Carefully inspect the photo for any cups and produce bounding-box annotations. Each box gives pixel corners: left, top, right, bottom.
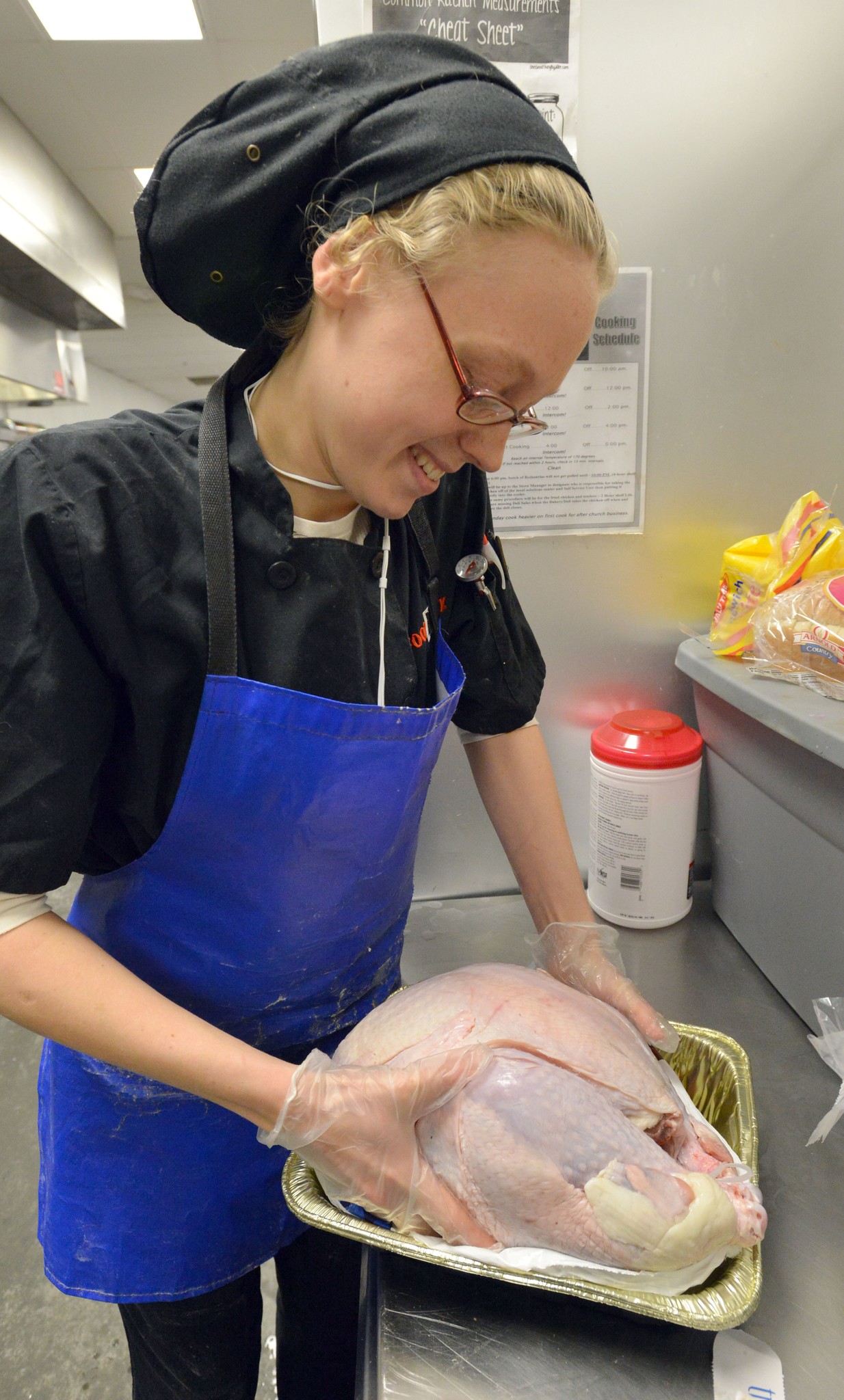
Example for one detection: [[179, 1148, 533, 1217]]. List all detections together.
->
[[584, 707, 704, 933]]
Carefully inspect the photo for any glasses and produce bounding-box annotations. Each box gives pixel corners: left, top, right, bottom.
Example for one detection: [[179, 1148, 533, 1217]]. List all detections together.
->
[[409, 253, 552, 441]]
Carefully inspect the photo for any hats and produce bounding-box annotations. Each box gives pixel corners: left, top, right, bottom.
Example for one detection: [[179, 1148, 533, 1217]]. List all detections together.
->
[[126, 30, 595, 346]]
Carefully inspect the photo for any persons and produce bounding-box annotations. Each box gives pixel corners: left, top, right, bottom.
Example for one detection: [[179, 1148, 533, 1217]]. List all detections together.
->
[[0, 30, 682, 1400]]
